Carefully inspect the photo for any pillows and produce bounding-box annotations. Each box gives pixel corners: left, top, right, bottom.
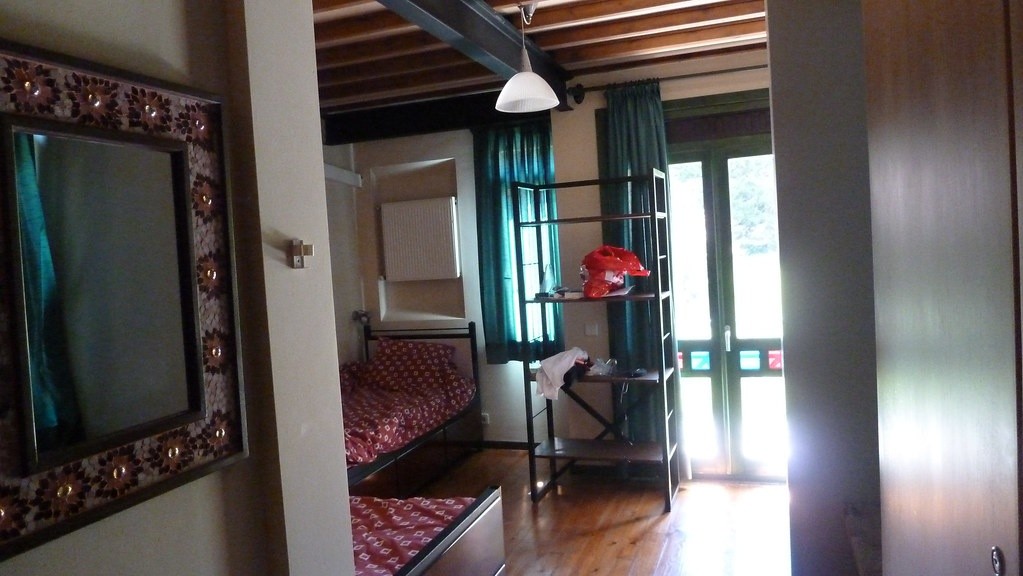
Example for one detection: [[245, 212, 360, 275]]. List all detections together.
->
[[362, 337, 458, 392]]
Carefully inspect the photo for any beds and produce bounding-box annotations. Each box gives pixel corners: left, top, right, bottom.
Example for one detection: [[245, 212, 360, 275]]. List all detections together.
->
[[339, 317, 483, 489], [348, 485, 506, 576]]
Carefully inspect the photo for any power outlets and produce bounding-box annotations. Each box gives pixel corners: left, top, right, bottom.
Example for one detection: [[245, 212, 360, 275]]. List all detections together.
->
[[481, 413, 489, 424]]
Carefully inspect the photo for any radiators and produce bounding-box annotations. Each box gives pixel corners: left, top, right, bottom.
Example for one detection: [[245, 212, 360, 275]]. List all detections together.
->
[[380, 197, 460, 281]]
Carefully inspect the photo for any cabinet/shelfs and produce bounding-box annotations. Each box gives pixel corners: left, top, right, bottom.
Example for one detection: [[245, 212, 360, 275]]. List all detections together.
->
[[509, 168, 679, 512]]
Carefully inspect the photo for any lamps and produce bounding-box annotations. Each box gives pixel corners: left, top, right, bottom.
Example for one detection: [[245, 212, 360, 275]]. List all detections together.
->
[[495, 6, 559, 113]]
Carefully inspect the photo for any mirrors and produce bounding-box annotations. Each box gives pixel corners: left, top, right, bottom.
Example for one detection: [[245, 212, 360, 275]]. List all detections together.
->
[[0, 37, 249, 563]]
